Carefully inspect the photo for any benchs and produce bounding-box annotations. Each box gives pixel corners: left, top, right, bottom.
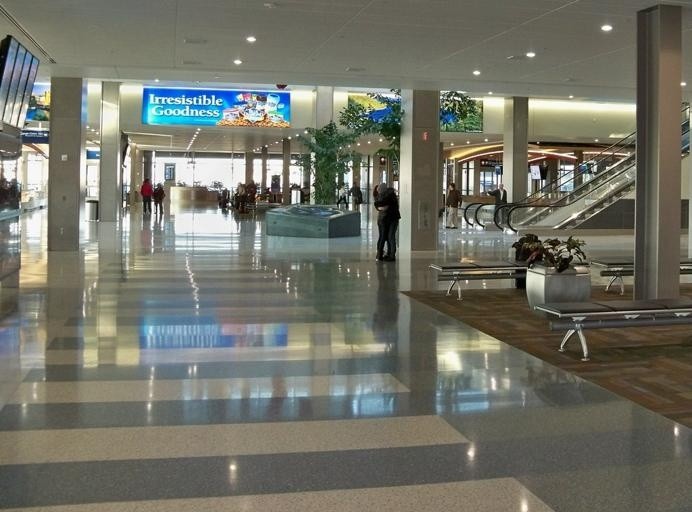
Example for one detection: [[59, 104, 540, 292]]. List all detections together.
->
[[429, 262, 532, 301], [230, 195, 283, 218], [533, 297, 692, 362], [590, 255, 692, 297]]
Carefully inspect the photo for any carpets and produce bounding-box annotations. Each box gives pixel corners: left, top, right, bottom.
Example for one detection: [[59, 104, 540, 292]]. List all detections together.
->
[[399, 283, 692, 429]]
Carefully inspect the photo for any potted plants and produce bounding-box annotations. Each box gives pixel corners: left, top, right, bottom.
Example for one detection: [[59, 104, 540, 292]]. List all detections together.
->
[[520, 235, 590, 311]]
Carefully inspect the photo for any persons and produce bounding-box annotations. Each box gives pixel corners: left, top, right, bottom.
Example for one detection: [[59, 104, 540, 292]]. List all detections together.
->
[[245, 179, 257, 199], [445, 183, 462, 229], [235, 182, 247, 211], [373, 184, 395, 262], [487, 184, 507, 224], [152, 183, 166, 214], [374, 182, 400, 262], [348, 182, 361, 212], [336, 182, 349, 207], [141, 178, 154, 215]]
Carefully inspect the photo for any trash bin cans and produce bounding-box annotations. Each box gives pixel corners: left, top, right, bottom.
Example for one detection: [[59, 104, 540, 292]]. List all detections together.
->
[[85, 200, 97, 221]]
